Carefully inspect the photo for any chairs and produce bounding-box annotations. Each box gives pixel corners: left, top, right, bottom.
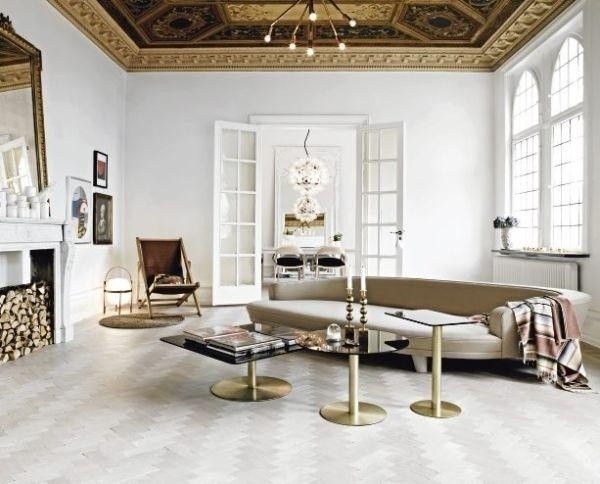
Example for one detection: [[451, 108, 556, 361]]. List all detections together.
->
[[272, 245, 304, 282], [136, 237, 202, 319], [312, 245, 347, 280]]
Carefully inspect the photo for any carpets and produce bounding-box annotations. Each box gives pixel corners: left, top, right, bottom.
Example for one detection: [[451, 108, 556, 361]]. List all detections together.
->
[[100, 312, 184, 329]]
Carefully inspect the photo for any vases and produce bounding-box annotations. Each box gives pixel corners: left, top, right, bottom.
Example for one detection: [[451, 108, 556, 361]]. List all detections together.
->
[[501, 230, 510, 250]]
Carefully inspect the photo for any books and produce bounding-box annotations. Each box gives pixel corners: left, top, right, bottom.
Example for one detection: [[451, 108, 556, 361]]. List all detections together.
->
[[184, 325, 308, 357]]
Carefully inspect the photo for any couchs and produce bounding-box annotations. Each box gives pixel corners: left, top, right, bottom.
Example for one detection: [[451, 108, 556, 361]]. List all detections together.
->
[[247, 276, 590, 372]]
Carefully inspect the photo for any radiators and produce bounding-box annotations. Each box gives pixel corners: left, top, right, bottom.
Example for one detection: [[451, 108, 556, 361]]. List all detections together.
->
[[492, 257, 578, 291]]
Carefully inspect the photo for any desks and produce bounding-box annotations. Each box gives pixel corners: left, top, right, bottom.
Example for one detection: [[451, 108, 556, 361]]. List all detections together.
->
[[386, 309, 479, 418], [296, 328, 410, 426]]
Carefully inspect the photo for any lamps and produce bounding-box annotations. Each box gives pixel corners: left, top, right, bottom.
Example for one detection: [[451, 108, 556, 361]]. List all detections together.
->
[[264, 1, 356, 56]]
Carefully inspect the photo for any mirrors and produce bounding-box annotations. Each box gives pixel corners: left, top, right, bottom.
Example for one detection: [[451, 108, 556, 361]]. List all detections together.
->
[[0, 27, 51, 217]]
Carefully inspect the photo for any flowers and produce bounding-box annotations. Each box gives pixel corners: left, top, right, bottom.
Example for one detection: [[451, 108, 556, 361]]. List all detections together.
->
[[494, 215, 519, 229]]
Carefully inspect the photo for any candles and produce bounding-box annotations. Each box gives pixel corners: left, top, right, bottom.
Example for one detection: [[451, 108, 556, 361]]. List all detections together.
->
[[346, 267, 352, 288], [360, 265, 366, 290]]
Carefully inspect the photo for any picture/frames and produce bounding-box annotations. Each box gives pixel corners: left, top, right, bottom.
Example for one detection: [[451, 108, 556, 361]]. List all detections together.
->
[[273, 145, 338, 249], [64, 177, 92, 243], [93, 192, 113, 244], [93, 150, 108, 188]]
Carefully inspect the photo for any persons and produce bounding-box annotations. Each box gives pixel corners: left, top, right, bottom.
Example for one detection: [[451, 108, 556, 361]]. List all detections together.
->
[[98, 202, 107, 235], [77, 197, 88, 237]]
